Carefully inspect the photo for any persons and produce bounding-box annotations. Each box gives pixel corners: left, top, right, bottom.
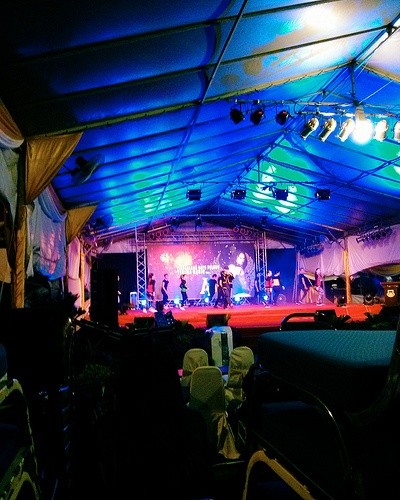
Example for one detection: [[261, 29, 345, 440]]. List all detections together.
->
[[180, 274, 189, 310], [208, 266, 234, 310], [147, 273, 157, 312], [264, 269, 287, 304], [295, 267, 308, 304], [161, 274, 169, 307], [254, 272, 261, 305], [314, 268, 325, 306]]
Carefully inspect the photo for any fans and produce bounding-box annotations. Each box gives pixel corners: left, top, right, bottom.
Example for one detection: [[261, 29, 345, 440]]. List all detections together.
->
[[76, 154, 103, 184]]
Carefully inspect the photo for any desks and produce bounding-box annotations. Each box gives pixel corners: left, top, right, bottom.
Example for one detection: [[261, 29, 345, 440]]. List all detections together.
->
[[243, 331, 397, 472]]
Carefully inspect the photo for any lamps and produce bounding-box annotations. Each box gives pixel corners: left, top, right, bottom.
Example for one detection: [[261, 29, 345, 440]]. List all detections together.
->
[[183, 181, 331, 201], [231, 100, 400, 143]]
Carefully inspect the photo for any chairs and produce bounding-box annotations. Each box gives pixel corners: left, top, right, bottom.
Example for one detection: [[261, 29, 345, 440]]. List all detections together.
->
[[179, 332, 255, 460]]
